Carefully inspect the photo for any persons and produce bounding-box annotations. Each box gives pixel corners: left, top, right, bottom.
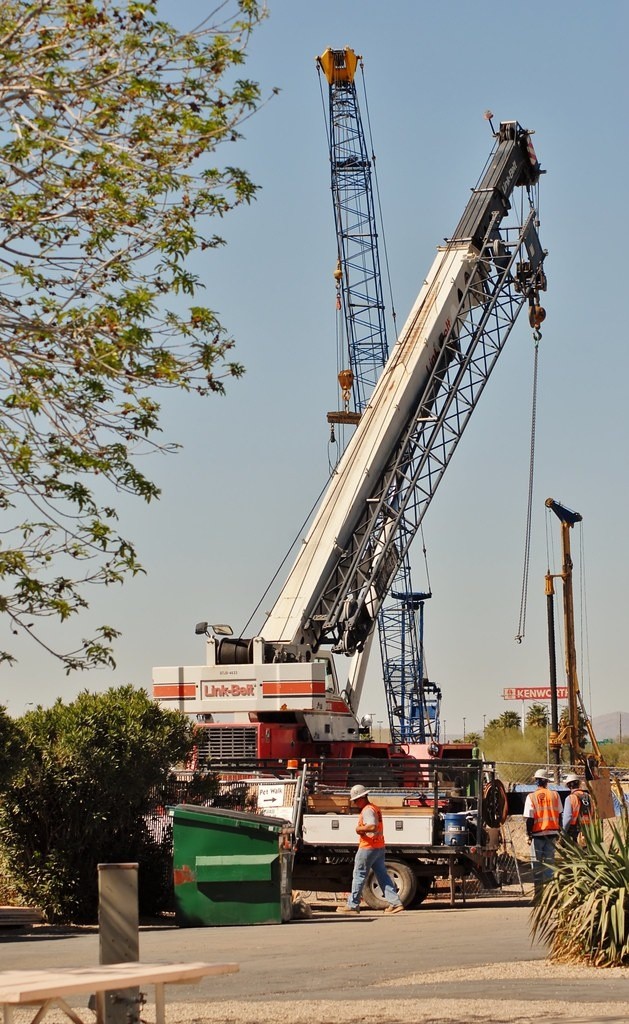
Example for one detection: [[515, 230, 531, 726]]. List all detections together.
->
[[336, 784, 404, 914], [523, 769, 564, 895], [557, 773, 596, 847]]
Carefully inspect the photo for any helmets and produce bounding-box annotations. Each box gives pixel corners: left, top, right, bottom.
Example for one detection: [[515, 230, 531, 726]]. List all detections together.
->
[[566, 775, 579, 784], [534, 769, 548, 779], [427, 741, 439, 756], [350, 785, 371, 801]]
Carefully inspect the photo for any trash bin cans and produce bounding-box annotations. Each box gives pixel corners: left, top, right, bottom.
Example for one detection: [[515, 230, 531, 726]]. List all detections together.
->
[[167, 799, 297, 925]]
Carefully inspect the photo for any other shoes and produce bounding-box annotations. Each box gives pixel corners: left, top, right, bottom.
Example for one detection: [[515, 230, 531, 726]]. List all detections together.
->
[[336, 906, 360, 914], [385, 905, 403, 914]]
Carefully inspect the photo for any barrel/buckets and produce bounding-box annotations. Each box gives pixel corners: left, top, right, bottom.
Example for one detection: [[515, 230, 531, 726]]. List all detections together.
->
[[445, 813, 470, 846]]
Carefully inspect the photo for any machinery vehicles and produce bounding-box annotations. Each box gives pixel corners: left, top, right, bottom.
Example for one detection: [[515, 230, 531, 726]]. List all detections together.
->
[[134, 42, 625, 911]]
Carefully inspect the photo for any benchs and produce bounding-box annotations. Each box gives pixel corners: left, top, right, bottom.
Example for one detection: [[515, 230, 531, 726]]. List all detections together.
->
[[0, 960, 240, 1024]]
